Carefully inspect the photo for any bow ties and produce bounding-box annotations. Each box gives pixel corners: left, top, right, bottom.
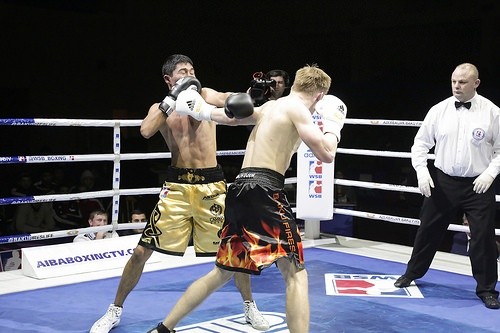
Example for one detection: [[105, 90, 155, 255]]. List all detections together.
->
[[455, 101, 471, 109]]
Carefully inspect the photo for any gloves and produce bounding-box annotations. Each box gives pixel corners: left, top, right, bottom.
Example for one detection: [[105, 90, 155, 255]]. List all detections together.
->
[[472, 167, 499, 194], [416, 167, 434, 197]]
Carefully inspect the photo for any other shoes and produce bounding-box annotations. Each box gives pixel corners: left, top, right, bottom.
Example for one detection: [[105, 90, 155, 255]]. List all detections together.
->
[[394, 272, 414, 288], [476, 289, 500, 309]]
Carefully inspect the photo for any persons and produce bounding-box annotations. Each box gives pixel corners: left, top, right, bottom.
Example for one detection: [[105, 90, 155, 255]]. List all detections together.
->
[[394, 63, 500, 309], [73, 211, 119, 242], [128, 209, 147, 234], [90, 56, 270, 333], [453, 213, 471, 257], [147, 66, 347, 333], [9, 169, 105, 232], [245, 69, 288, 100]]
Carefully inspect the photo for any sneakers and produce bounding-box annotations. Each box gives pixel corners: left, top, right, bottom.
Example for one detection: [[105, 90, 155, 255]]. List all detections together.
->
[[90, 304, 123, 333], [243, 300, 269, 331]]
